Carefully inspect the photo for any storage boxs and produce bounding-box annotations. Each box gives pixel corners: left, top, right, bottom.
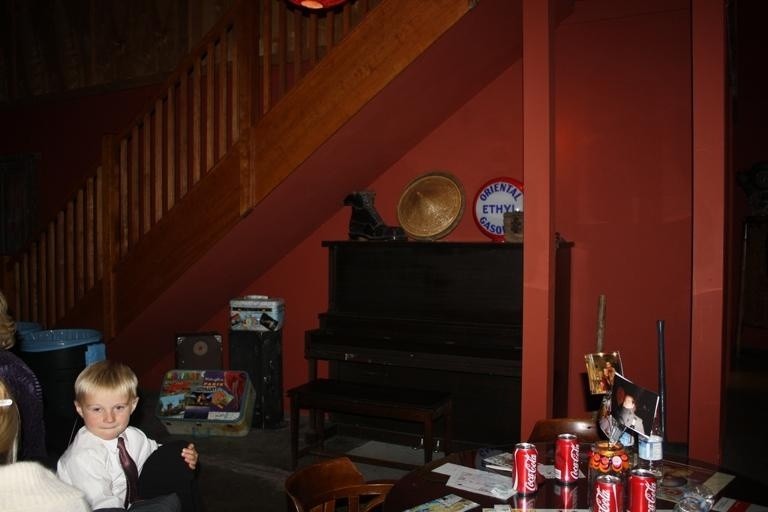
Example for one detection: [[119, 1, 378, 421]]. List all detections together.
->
[[155, 369, 257, 438], [230, 294, 285, 332]]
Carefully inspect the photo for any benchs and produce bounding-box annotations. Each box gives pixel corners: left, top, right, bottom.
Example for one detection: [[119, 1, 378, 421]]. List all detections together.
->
[[286, 378, 456, 471]]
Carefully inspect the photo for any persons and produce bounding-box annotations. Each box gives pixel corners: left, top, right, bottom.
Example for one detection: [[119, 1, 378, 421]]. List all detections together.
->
[[56, 357, 202, 512], [617, 392, 646, 435], [0, 376, 94, 512], [1, 291, 50, 460], [602, 360, 616, 391]]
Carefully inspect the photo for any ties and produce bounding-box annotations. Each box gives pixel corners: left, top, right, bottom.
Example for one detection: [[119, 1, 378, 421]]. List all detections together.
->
[[117, 437, 140, 509]]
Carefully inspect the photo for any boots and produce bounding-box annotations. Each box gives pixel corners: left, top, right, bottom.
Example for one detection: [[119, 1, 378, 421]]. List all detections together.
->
[[343, 191, 408, 241]]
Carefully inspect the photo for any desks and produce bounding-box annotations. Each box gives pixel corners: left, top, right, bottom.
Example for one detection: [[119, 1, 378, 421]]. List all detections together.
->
[[382, 440, 768, 512]]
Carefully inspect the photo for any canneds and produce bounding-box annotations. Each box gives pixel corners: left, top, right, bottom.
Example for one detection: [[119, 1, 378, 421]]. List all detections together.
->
[[592, 475, 624, 512], [512, 442, 538, 497], [554, 434, 580, 485], [626, 469, 656, 512]]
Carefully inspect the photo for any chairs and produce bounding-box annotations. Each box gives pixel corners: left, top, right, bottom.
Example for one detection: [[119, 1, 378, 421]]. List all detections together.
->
[[285, 457, 396, 512]]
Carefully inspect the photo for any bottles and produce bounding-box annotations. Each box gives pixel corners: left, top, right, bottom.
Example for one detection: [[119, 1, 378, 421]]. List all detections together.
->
[[638, 417, 665, 483]]
